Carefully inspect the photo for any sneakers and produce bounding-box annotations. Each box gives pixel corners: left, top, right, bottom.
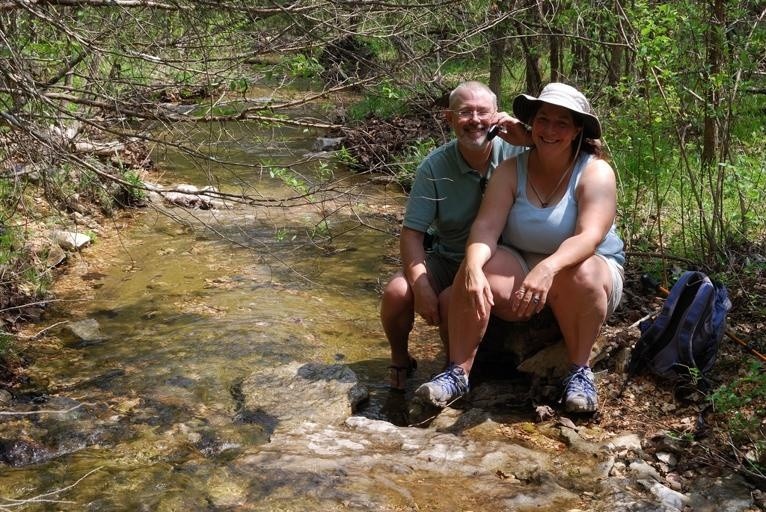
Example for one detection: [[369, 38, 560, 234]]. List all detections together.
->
[[563, 364, 598, 412], [413, 366, 471, 407], [388, 358, 417, 387]]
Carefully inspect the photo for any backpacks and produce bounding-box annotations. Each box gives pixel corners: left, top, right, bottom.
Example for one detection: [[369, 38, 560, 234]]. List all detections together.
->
[[630, 271, 734, 398]]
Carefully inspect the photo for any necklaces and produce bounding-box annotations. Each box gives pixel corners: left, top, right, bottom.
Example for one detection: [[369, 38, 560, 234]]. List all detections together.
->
[[460, 141, 493, 197]]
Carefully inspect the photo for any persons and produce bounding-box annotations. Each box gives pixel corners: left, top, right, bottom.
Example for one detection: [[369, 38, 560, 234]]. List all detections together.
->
[[374, 81, 539, 402], [414, 80, 627, 414]]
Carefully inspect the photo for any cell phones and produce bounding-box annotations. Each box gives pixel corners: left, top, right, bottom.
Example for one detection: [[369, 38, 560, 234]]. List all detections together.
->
[[487, 124, 502, 141]]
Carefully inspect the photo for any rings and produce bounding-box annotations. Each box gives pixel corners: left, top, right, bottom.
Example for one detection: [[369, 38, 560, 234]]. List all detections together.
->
[[533, 296, 540, 305], [433, 320, 439, 324], [520, 287, 526, 296]]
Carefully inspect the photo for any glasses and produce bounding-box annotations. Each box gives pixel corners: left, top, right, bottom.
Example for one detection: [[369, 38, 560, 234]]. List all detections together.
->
[[450, 109, 495, 119]]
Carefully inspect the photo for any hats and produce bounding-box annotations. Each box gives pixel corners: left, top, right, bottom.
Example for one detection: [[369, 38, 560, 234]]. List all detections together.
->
[[514, 82, 602, 139]]
